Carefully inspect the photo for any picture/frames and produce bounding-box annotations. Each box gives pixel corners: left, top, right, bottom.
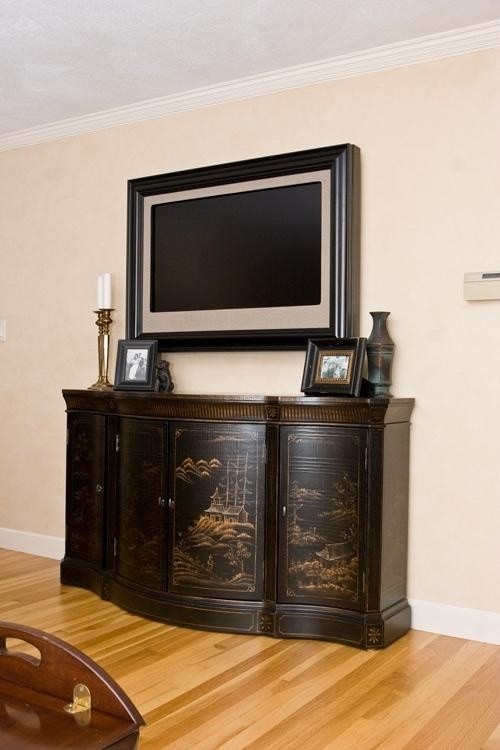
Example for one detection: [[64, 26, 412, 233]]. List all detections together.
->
[[113, 339, 160, 392], [124, 143, 360, 351], [301, 336, 368, 398]]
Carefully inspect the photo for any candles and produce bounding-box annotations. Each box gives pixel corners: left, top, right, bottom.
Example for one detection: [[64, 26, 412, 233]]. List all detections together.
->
[[95, 271, 112, 311]]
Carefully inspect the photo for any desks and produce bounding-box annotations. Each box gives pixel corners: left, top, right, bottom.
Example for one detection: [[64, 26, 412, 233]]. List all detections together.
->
[[0, 620, 146, 750]]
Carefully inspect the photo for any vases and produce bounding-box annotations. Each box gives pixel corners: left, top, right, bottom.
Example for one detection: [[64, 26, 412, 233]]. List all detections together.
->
[[367, 311, 396, 398]]
